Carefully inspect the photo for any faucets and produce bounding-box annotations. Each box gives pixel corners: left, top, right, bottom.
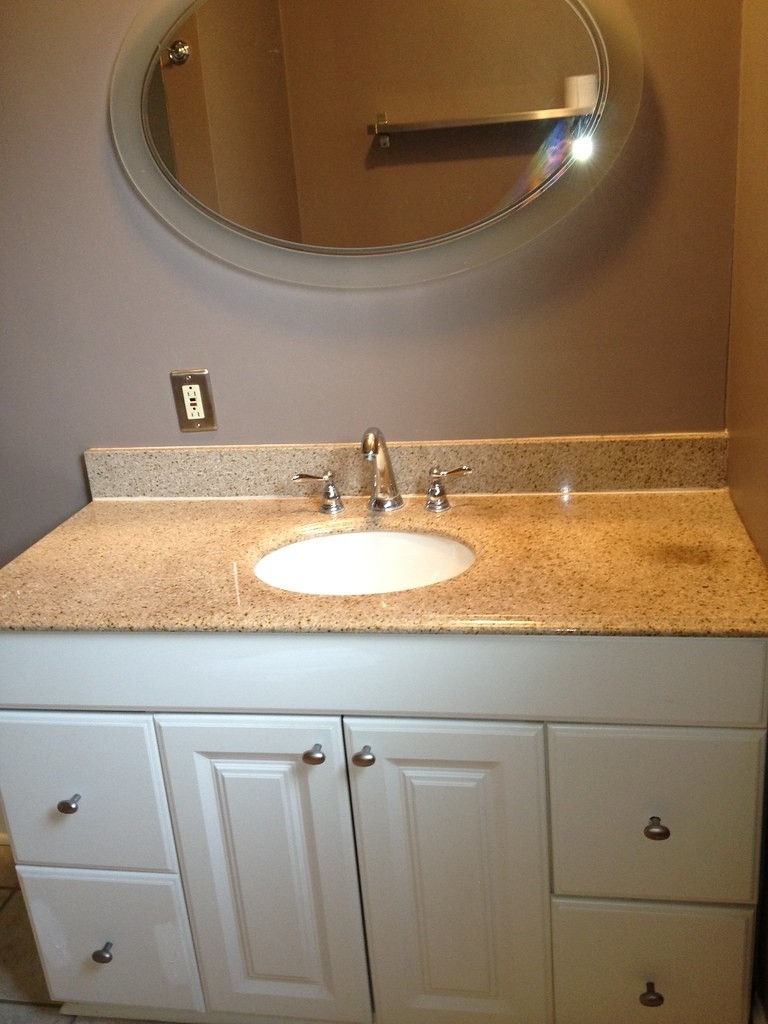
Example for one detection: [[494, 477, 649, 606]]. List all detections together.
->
[[361, 426, 404, 511]]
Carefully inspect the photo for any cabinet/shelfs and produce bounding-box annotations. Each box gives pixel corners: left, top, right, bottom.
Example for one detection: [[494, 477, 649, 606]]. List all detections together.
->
[[0, 628, 207, 1024], [154, 632, 556, 1024], [543, 633, 768, 1024]]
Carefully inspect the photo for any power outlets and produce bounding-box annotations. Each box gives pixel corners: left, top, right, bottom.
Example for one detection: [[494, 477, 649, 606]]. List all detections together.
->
[[170, 368, 218, 433]]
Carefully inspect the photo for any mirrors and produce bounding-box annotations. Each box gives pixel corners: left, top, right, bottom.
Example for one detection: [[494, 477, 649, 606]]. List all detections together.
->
[[109, 0, 645, 289]]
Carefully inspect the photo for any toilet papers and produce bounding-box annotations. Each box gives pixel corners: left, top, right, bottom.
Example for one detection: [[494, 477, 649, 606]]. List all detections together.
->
[[564, 74, 597, 108]]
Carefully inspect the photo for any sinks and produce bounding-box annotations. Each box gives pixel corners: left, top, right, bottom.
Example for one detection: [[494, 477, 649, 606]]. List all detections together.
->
[[254, 531, 475, 597]]
